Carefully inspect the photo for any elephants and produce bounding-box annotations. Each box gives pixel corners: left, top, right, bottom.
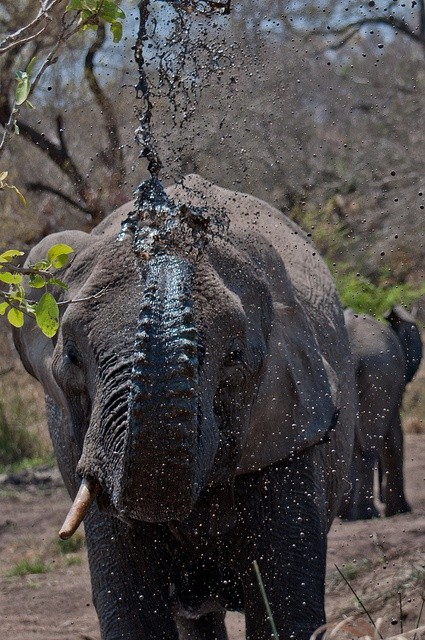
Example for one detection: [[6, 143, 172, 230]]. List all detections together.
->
[[339, 306, 423, 520], [8, 174, 356, 640]]
